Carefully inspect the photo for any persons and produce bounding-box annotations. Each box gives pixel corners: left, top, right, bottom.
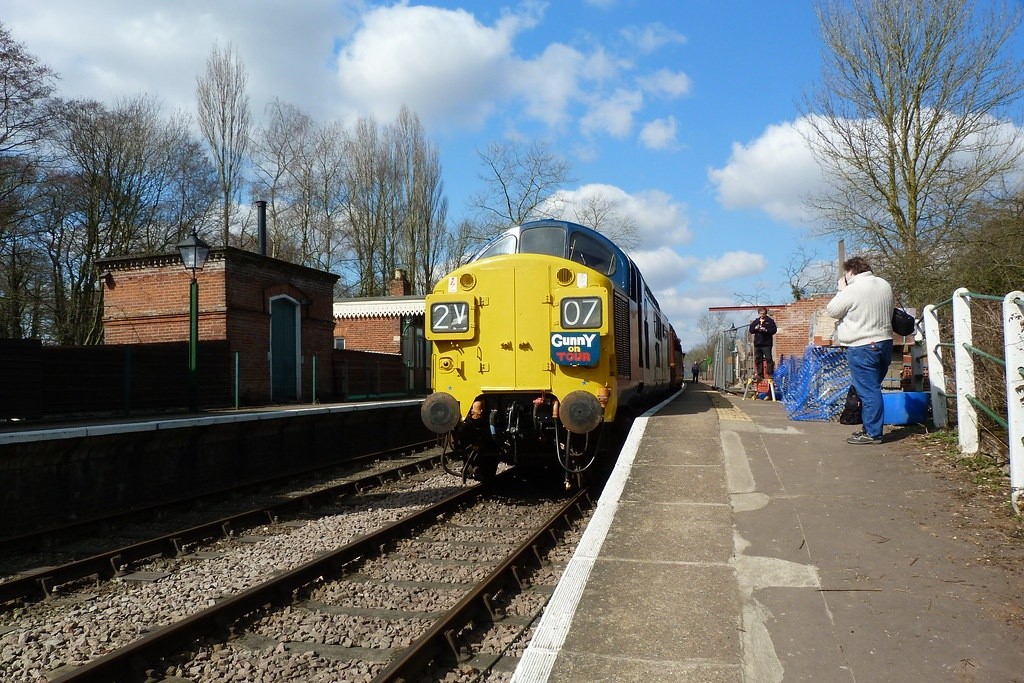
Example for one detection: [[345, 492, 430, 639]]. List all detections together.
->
[[828, 257, 894, 443], [692, 360, 699, 384], [749, 307, 777, 378]]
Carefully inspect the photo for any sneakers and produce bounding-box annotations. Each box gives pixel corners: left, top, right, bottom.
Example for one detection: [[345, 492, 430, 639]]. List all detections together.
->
[[852, 430, 868, 436], [848, 434, 882, 444]]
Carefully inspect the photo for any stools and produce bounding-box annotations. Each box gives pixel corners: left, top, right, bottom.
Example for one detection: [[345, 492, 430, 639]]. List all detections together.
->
[[742, 378, 778, 402]]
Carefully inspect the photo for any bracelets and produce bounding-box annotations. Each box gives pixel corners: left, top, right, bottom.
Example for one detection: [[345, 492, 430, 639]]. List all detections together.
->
[[766, 330, 767, 332]]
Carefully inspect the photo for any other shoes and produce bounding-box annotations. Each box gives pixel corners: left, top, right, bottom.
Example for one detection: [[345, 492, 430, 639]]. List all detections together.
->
[[753, 375, 763, 380], [769, 374, 774, 379]]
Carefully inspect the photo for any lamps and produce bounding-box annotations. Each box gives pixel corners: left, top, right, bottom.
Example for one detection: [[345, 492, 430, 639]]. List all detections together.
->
[[99, 271, 110, 283]]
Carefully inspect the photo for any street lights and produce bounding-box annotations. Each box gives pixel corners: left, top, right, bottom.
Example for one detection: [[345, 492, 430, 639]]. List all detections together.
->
[[727, 323, 737, 387], [176, 225, 212, 416]]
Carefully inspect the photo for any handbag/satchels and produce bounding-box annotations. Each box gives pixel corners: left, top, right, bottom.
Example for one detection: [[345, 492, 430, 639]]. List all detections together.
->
[[891, 308, 915, 336]]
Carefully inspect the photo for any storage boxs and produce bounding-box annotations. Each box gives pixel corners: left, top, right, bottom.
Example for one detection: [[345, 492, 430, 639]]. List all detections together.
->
[[881, 392, 931, 425], [881, 350, 904, 388]]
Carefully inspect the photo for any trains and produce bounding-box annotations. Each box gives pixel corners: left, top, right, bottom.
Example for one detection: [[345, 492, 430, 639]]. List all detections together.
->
[[422, 219, 685, 491]]
